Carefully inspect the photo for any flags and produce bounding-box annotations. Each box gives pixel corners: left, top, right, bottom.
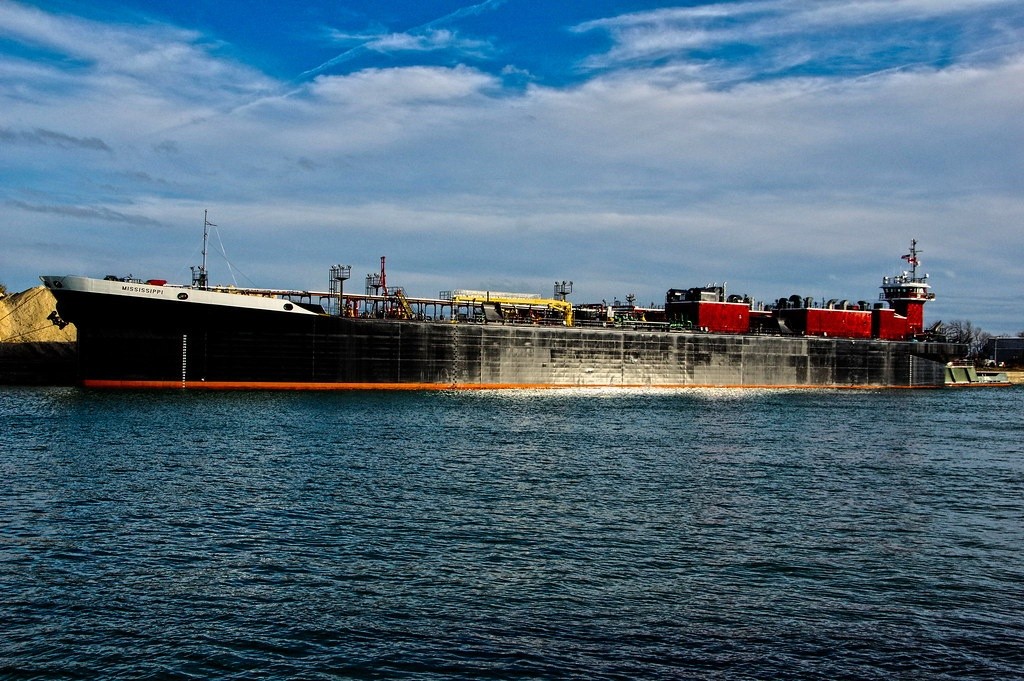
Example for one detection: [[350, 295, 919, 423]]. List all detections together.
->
[[901, 254, 916, 263]]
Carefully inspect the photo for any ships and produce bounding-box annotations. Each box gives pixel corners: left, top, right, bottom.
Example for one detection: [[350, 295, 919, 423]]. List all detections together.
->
[[39, 205, 969, 391]]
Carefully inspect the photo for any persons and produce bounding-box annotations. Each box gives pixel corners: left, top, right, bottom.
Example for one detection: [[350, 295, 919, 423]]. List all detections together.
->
[[348, 304, 354, 317], [946, 356, 968, 366], [508, 308, 565, 326], [360, 311, 398, 320]]
